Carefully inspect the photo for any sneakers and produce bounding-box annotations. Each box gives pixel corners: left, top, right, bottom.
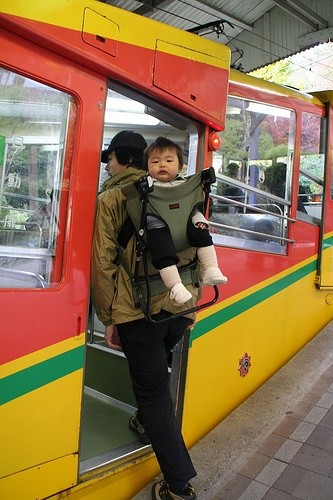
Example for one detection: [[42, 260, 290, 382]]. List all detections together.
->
[[151, 479, 197, 500], [129, 410, 151, 445]]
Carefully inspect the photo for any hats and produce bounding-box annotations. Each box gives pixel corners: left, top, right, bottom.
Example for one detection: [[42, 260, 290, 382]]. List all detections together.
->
[[101, 131, 147, 163]]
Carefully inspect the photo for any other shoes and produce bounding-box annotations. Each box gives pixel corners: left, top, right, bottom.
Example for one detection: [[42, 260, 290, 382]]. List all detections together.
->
[[170, 283, 192, 303], [203, 267, 227, 285]]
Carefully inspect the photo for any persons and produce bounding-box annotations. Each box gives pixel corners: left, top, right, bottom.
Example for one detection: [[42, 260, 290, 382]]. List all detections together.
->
[[263, 163, 307, 214], [25, 190, 60, 250], [91, 130, 204, 500], [218, 163, 246, 205], [143, 136, 227, 304]]
